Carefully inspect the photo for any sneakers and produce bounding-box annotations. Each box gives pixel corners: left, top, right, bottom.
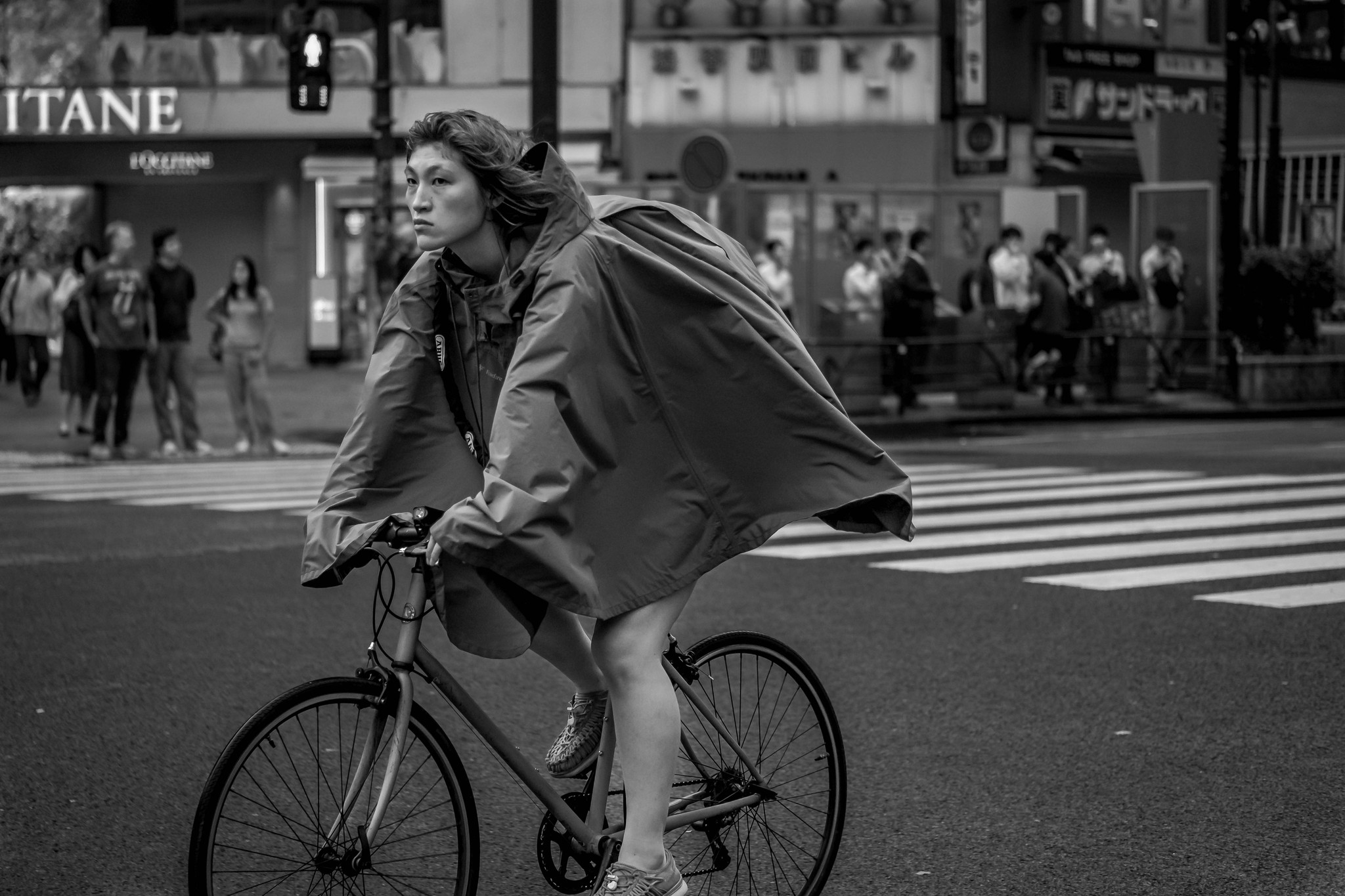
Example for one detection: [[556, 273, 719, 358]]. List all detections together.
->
[[593, 849, 688, 896], [545, 688, 608, 778]]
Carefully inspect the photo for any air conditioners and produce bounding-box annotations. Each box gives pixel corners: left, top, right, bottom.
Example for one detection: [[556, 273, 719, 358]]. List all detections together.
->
[[956, 116, 1006, 161]]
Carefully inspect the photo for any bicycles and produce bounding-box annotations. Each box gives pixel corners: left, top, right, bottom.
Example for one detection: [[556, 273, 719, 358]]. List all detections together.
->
[[189, 505, 848, 896]]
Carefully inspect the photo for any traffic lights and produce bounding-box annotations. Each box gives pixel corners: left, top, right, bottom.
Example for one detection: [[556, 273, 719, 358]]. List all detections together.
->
[[289, 29, 334, 115]]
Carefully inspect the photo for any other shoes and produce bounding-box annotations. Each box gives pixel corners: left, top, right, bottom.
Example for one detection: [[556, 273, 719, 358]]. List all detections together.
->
[[24, 392, 39, 403], [77, 425, 91, 435], [897, 399, 929, 413], [194, 439, 214, 454], [89, 443, 112, 461], [59, 422, 69, 437], [236, 438, 250, 453], [164, 439, 176, 455], [112, 445, 139, 459], [1043, 395, 1084, 407], [272, 439, 291, 454]]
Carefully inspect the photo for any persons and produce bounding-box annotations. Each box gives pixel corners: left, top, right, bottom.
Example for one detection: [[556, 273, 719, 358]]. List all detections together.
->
[[0, 219, 211, 460], [202, 254, 294, 457], [1140, 225, 1188, 391], [756, 223, 1127, 418], [299, 109, 729, 896]]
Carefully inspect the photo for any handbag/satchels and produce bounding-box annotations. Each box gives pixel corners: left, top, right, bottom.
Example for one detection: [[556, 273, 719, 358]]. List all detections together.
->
[[208, 286, 229, 361]]
